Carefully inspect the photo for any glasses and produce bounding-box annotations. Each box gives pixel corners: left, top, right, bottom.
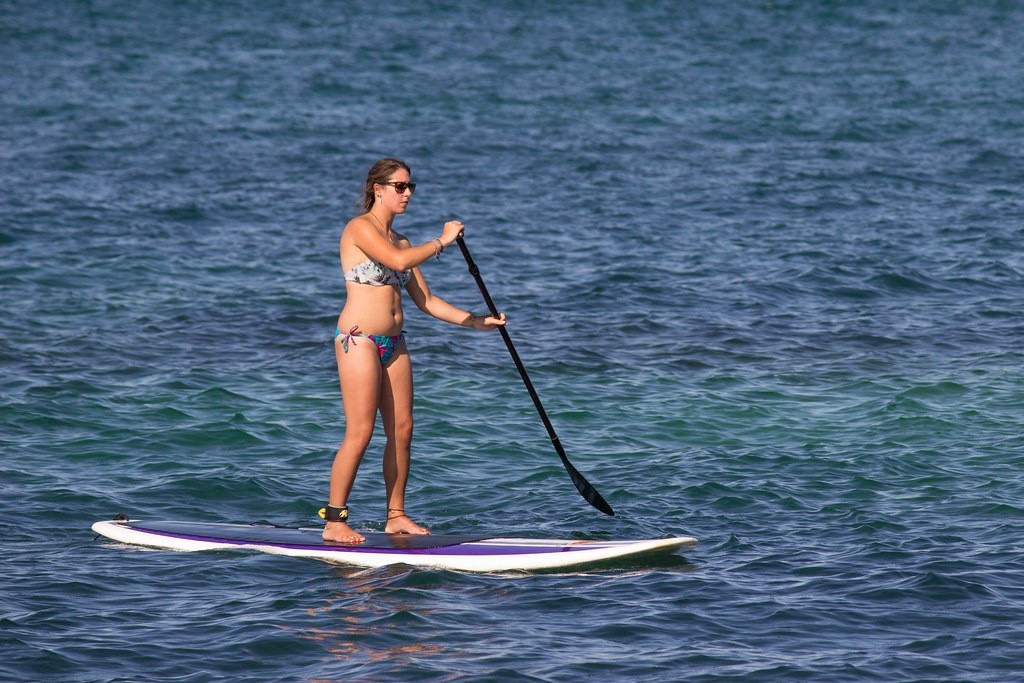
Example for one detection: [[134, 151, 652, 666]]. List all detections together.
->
[[378, 181, 416, 194]]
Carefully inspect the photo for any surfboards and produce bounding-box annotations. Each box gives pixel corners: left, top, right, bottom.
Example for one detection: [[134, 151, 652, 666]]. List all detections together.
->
[[88, 515, 700, 574]]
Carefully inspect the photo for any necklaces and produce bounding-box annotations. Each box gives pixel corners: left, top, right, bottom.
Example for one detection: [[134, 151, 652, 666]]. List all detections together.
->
[[369, 211, 394, 244]]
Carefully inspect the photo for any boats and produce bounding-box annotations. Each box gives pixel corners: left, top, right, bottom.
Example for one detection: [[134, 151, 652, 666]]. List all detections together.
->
[[92, 513, 699, 570]]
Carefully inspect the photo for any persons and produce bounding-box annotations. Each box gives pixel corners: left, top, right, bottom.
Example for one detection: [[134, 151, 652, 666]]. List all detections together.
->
[[320, 156, 508, 544]]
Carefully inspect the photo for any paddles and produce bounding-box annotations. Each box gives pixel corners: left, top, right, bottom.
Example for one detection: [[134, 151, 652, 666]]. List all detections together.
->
[[456, 233, 617, 517]]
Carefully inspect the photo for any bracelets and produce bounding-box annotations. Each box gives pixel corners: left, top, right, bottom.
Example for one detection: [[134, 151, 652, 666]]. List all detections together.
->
[[436, 238, 444, 253], [431, 240, 441, 259], [471, 315, 476, 328]]
